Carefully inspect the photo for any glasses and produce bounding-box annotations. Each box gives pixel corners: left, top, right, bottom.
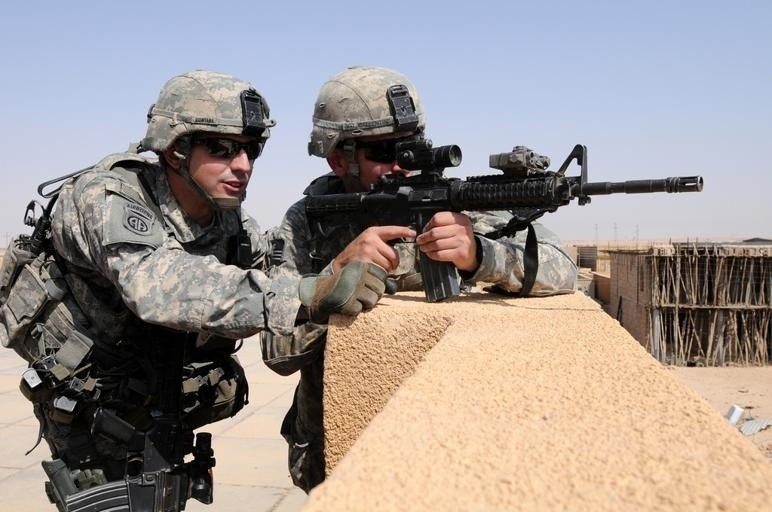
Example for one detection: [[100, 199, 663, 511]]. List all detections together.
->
[[190, 136, 266, 160], [336, 127, 424, 164]]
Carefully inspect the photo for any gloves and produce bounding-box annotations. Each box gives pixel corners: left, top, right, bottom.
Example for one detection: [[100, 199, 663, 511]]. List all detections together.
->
[[298, 262, 398, 323]]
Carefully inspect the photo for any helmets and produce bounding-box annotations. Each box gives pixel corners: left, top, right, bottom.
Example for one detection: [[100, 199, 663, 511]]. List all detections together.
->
[[308, 67, 425, 157], [140, 70, 277, 153]]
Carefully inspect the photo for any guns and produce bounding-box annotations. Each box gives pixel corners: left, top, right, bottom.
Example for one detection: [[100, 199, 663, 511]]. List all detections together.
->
[[305, 136, 703, 303], [65, 313, 215, 512]]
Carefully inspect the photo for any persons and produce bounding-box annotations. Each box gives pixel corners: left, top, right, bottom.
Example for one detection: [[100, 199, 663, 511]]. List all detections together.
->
[[258, 65, 581, 495], [2, 69, 399, 512]]
